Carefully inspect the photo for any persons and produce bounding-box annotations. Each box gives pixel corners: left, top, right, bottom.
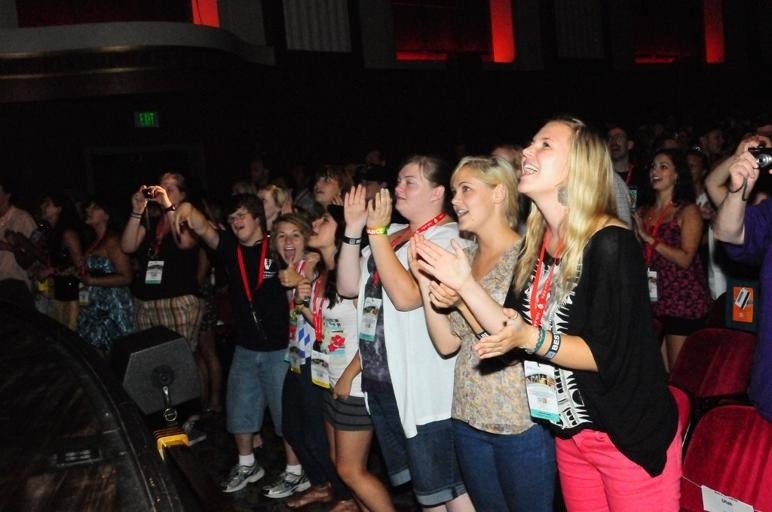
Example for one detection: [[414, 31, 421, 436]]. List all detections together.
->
[[652, 125, 772, 208], [408, 156, 557, 511], [0, 187, 38, 299], [603, 123, 646, 230], [217, 151, 409, 224], [170, 192, 311, 497], [195, 203, 225, 415], [336, 156, 477, 512], [33, 192, 84, 332], [711, 139, 772, 428], [270, 214, 359, 512], [296, 205, 395, 512], [412, 119, 682, 507], [77, 195, 139, 361], [224, 151, 398, 233], [632, 151, 711, 374], [488, 144, 532, 237], [121, 172, 207, 354], [687, 153, 731, 329]]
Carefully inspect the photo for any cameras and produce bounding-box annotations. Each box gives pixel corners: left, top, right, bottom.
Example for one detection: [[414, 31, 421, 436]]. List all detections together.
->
[[750, 146, 768, 168], [143, 188, 159, 199]]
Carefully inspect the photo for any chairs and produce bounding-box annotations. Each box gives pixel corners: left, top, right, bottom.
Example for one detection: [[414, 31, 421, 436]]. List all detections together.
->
[[680, 405, 772, 512], [668, 385, 691, 448], [667, 327, 755, 397]]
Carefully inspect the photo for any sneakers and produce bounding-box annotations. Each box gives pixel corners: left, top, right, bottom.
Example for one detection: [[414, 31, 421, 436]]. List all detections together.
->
[[264, 470, 311, 498], [220, 462, 264, 492]]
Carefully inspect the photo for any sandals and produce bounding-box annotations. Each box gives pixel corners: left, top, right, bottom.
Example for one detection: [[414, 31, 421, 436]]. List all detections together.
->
[[328, 499, 359, 512], [285, 484, 332, 507]]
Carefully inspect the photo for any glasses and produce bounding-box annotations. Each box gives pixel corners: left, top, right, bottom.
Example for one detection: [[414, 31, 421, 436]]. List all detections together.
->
[[226, 213, 252, 224]]
